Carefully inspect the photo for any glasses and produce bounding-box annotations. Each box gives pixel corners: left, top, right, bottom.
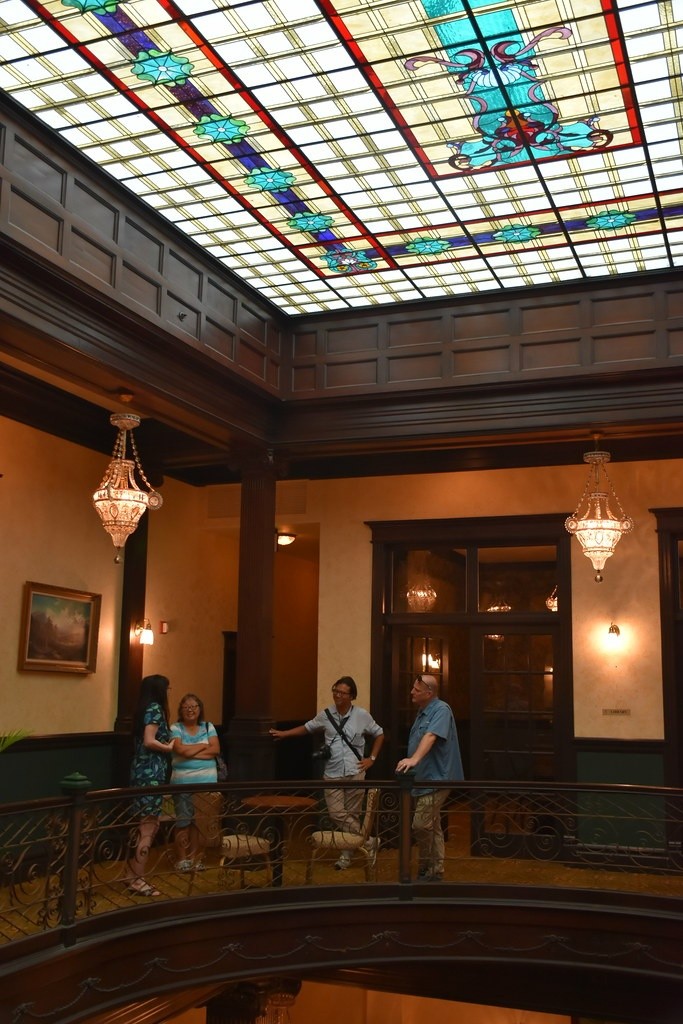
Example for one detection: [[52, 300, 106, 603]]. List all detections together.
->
[[415, 674, 429, 687], [332, 688, 351, 696], [182, 705, 200, 710], [167, 687, 173, 693]]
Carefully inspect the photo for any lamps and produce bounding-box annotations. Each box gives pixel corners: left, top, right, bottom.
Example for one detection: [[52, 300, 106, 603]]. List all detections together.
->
[[563, 431, 634, 584], [134, 618, 154, 645], [544, 585, 557, 611], [91, 394, 164, 563], [605, 623, 620, 651], [407, 551, 437, 612], [485, 593, 512, 612], [278, 533, 297, 545]]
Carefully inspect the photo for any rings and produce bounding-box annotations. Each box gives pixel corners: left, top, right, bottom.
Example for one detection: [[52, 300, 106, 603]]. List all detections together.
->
[[403, 764, 406, 767]]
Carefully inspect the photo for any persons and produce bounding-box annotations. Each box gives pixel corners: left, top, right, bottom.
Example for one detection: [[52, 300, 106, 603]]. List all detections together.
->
[[166, 694, 221, 872], [396, 674, 464, 880], [128, 674, 176, 896], [269, 675, 385, 870]]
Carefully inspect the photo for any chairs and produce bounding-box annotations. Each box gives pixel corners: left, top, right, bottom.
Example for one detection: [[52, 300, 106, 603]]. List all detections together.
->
[[304, 788, 380, 884], [186, 792, 270, 897]]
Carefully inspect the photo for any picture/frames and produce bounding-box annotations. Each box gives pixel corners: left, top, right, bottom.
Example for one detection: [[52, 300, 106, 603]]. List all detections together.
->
[[17, 580, 102, 674]]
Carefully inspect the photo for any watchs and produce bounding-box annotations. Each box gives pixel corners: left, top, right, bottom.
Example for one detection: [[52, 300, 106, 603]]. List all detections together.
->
[[370, 756, 375, 761]]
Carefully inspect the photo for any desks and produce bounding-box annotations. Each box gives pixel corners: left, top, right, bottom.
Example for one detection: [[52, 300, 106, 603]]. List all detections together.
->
[[240, 796, 319, 886]]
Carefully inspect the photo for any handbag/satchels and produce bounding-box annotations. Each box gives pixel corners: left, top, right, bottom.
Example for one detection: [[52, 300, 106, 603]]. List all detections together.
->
[[205, 721, 228, 780]]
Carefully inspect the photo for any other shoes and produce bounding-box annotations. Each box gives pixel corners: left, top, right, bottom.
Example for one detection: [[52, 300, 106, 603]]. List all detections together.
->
[[417, 868, 431, 881], [335, 856, 349, 868], [433, 874, 443, 881], [360, 836, 381, 868], [179, 858, 204, 871]]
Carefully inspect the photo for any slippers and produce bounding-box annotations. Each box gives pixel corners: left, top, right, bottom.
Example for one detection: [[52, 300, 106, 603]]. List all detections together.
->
[[128, 885, 161, 896]]
